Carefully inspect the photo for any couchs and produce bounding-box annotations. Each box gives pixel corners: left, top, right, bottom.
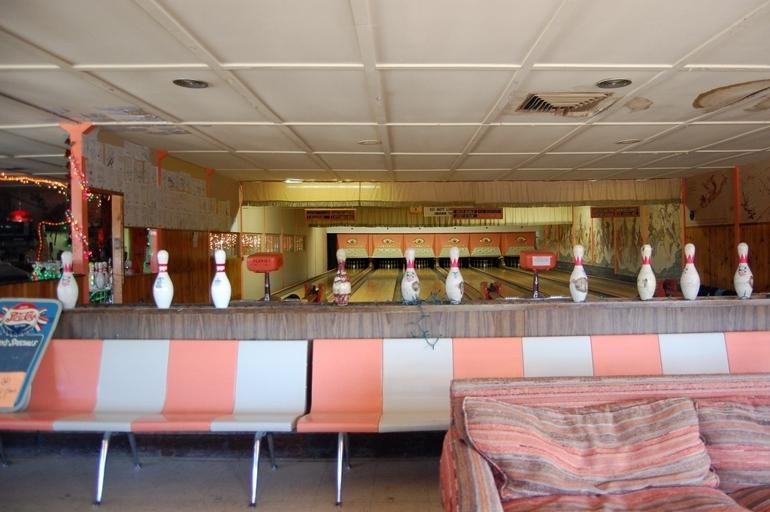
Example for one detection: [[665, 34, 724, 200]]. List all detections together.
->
[[439, 373, 770, 512]]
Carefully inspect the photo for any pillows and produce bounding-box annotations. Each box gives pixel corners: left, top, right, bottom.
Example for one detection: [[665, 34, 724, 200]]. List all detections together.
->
[[695, 397, 769, 494], [463, 396, 720, 499]]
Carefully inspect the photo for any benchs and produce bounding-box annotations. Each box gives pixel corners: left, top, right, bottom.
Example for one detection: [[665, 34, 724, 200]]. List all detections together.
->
[[0, 340, 308, 507], [296, 331, 770, 504]]
